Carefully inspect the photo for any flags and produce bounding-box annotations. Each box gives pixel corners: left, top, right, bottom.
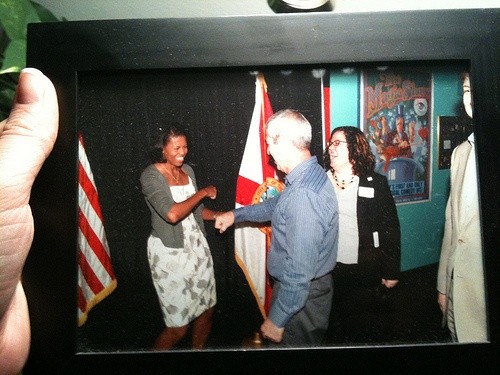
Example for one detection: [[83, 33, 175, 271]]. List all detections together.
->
[[77, 131, 118, 326], [235, 75, 289, 321]]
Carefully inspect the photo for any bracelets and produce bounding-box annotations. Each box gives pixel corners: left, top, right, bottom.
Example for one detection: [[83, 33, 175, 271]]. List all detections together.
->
[[213, 211, 218, 221]]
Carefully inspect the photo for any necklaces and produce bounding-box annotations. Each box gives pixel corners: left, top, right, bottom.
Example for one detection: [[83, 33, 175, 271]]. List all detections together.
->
[[160, 162, 180, 185], [333, 171, 356, 189]]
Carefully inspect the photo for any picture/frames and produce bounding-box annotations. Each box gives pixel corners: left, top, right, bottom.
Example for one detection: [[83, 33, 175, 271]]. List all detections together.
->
[[23, 7, 500, 375], [360, 64, 434, 206]]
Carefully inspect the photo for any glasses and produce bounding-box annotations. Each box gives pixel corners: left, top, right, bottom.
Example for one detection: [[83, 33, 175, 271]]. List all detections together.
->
[[326, 140, 349, 146]]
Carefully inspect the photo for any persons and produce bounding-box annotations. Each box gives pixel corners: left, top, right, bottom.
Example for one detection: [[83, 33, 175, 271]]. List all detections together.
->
[[321, 126, 402, 347], [0, 67, 61, 374], [138, 124, 224, 350], [436, 70, 490, 344], [213, 108, 340, 349]]
